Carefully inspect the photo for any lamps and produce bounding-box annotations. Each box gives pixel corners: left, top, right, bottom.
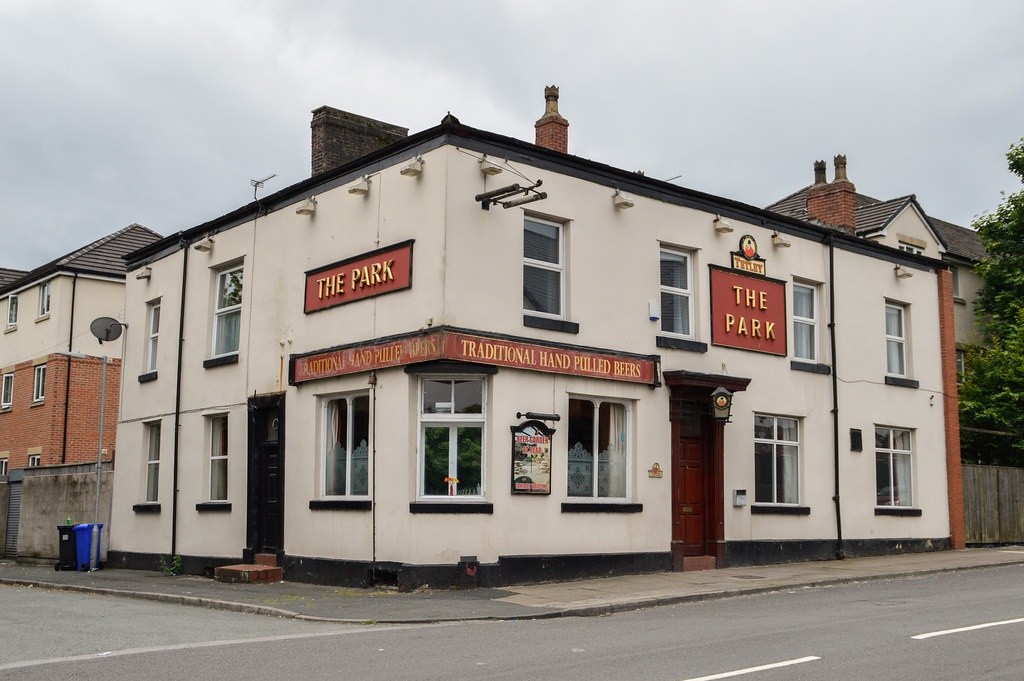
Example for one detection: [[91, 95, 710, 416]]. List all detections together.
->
[[894, 262, 912, 280], [401, 153, 425, 177], [612, 188, 634, 208], [296, 195, 317, 215], [478, 153, 502, 176], [713, 214, 733, 233], [771, 230, 791, 247], [348, 174, 372, 195], [194, 235, 215, 252], [136, 267, 152, 279], [711, 387, 734, 424]]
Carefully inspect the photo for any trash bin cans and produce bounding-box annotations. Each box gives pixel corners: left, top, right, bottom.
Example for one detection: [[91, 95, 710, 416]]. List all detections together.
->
[[72, 522, 104, 572], [54, 524, 79, 571]]
